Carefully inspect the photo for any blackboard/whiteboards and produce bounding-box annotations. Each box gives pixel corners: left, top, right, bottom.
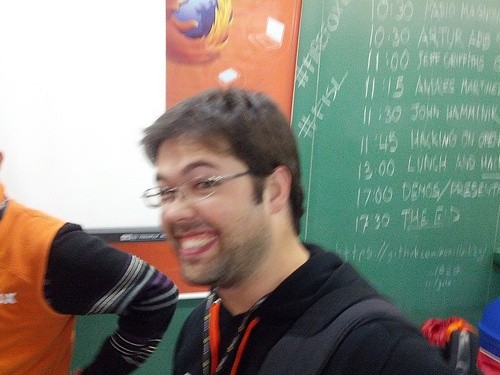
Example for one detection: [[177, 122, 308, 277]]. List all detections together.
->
[[289, 0, 500, 336], [69, 292, 211, 375], [0, 1, 168, 242]]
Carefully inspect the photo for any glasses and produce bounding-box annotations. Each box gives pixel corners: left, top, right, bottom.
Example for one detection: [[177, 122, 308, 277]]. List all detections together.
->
[[141, 170, 251, 207]]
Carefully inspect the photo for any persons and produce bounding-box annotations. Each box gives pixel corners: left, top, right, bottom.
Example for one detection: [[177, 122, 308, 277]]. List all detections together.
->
[[1, 152, 180, 375], [138, 87, 455, 375]]
